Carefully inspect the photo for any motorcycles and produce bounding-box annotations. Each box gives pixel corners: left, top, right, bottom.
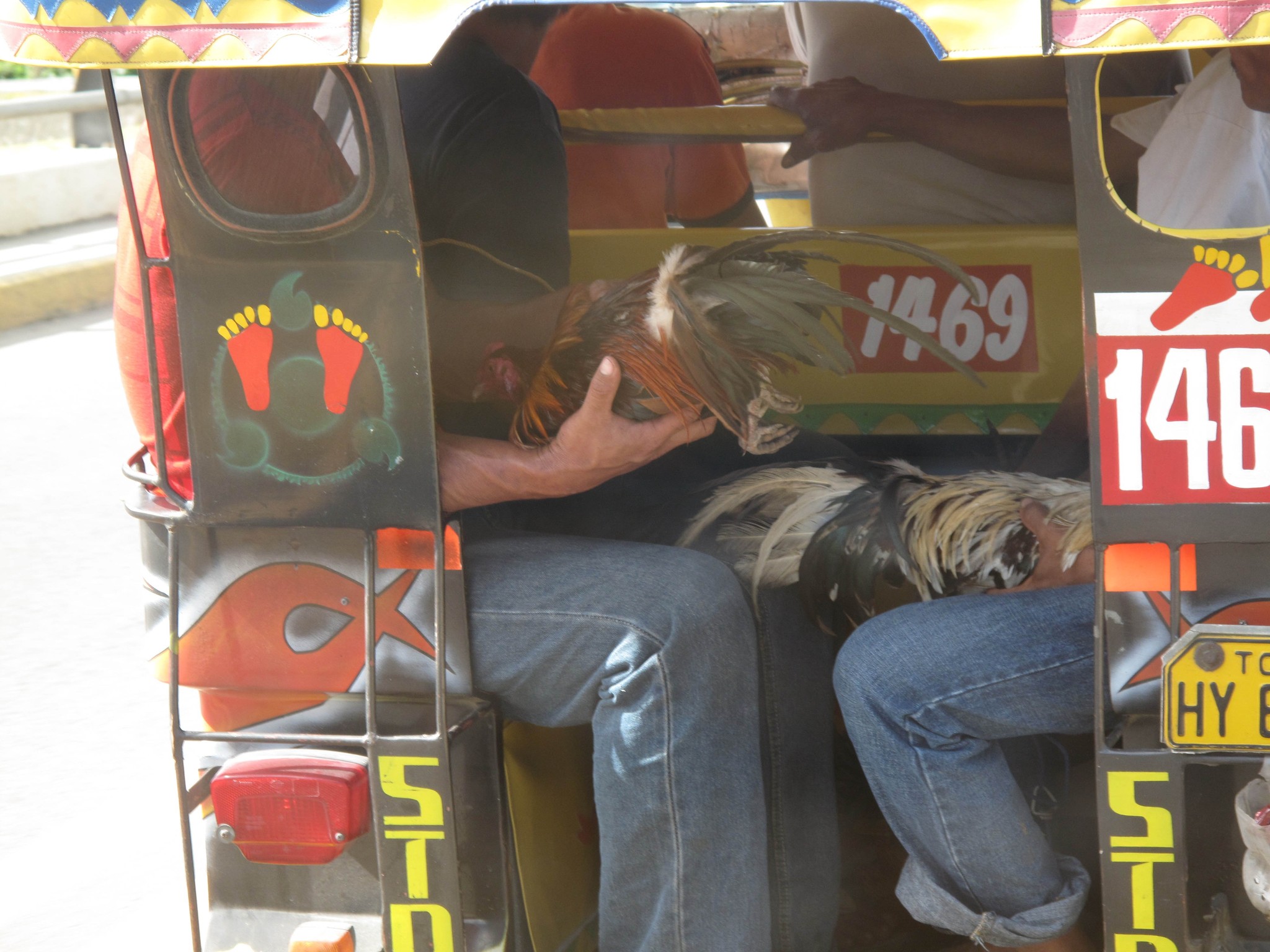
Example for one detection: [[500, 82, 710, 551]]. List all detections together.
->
[[18, 0, 1269, 951]]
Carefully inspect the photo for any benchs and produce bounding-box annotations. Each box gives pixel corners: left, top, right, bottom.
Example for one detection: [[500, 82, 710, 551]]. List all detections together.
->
[[202, 690, 600, 952]]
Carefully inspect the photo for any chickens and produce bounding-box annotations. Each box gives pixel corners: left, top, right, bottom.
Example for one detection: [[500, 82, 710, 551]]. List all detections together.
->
[[475, 230, 991, 455], [676, 456, 1096, 618]]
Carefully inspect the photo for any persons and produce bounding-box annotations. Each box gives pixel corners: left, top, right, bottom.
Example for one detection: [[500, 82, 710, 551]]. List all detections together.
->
[[430, 0, 769, 231], [392, 37, 569, 301], [108, 45, 845, 950], [767, 45, 1270, 951]]
